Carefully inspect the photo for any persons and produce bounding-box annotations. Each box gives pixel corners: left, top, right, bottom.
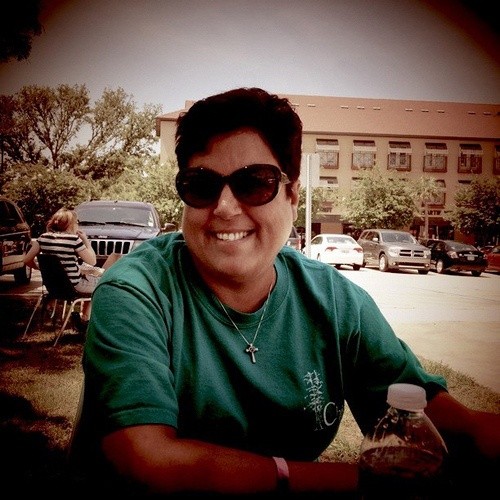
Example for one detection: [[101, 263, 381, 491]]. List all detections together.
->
[[62, 86, 499, 499], [24, 207, 100, 331]]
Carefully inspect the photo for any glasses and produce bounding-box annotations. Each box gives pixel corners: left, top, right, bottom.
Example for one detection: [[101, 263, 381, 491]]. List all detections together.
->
[[175, 164, 293, 207]]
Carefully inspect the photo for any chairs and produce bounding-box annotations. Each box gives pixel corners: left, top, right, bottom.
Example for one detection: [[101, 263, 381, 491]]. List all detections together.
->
[[23, 252, 124, 348]]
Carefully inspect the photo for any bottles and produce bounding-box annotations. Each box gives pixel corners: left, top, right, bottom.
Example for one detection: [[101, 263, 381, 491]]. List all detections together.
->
[[355, 383, 448, 500]]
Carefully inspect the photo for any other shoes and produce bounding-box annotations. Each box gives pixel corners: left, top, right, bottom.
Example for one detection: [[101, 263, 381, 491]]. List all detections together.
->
[[71, 312, 83, 331], [81, 321, 89, 345]]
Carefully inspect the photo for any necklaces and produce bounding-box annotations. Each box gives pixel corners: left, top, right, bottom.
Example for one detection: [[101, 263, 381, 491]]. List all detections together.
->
[[214, 273, 273, 364]]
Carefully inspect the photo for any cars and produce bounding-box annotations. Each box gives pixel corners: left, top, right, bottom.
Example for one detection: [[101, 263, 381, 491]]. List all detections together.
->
[[427, 239, 500, 276], [302, 234, 363, 271], [72, 200, 175, 260], [0, 196, 32, 286]]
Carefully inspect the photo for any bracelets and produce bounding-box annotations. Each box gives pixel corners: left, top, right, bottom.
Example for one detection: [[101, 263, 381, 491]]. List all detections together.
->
[[274, 456, 289, 496]]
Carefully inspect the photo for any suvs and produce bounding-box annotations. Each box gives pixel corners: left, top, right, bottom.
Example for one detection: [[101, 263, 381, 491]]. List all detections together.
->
[[356, 230, 431, 275]]
